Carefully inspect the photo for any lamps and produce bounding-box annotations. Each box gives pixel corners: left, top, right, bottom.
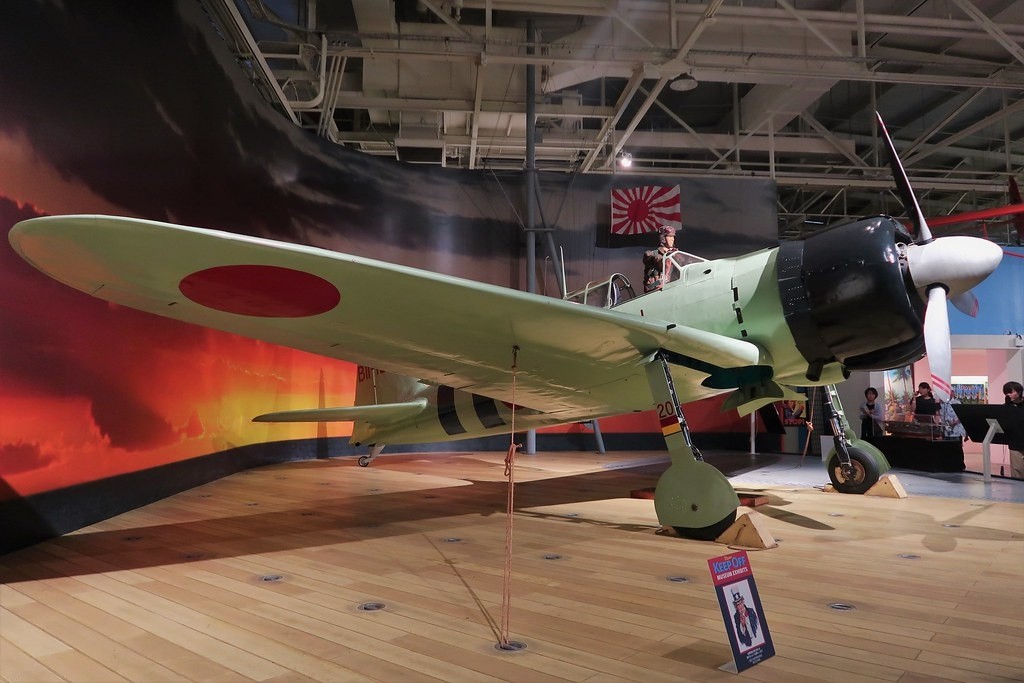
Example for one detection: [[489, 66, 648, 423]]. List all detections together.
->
[[669, 73, 699, 91]]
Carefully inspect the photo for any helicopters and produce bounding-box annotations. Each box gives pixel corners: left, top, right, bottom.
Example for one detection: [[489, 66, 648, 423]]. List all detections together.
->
[[6, 107, 1004, 542]]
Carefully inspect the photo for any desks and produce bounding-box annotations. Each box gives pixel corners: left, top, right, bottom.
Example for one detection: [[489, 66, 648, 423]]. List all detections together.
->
[[864, 435, 966, 473]]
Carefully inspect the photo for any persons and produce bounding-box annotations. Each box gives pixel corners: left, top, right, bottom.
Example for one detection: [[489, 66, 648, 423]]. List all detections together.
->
[[643, 226, 689, 291], [910, 382, 941, 424], [860, 388, 885, 436], [731, 585, 758, 646], [783, 400, 804, 419], [1003, 381, 1024, 404]]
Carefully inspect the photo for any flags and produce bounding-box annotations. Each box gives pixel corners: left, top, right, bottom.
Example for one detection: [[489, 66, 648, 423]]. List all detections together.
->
[[610, 184, 682, 234]]
